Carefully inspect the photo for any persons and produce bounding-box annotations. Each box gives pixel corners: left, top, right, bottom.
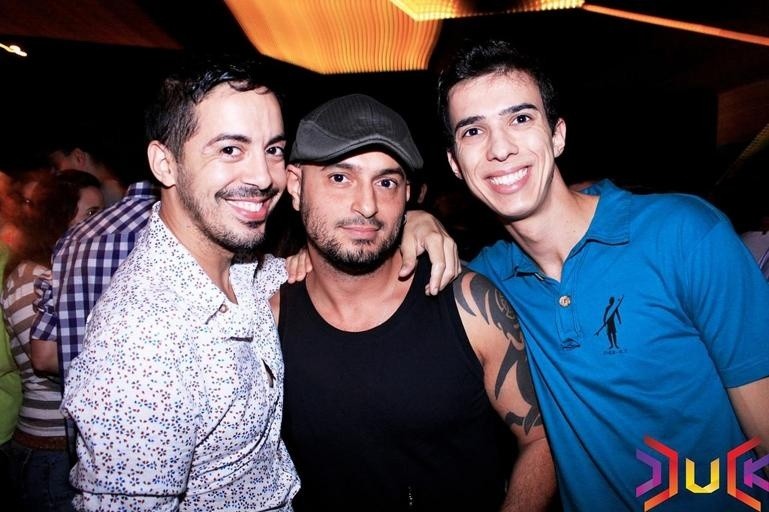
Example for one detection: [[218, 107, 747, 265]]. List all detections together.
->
[[270, 94, 558, 512], [283, 41, 769, 512], [0, 133, 162, 512], [59, 58, 463, 512]]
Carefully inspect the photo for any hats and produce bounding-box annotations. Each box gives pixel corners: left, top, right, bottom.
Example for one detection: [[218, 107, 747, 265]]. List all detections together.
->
[[288, 96, 424, 171]]
